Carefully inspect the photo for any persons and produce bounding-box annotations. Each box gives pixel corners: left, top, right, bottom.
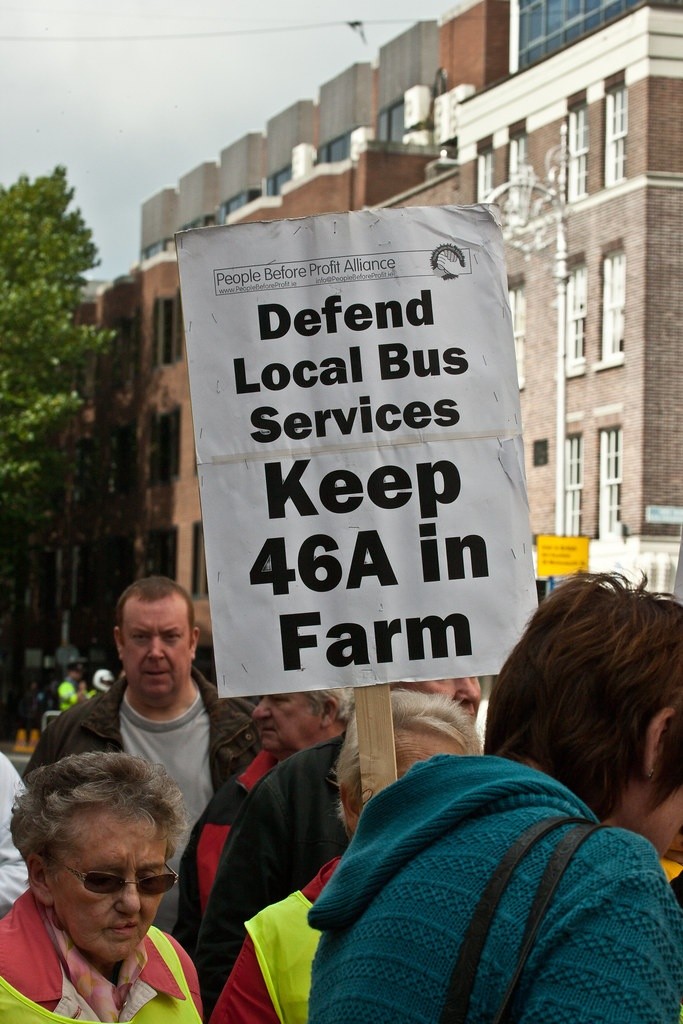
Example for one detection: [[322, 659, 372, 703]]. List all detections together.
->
[[0, 570, 683, 1023]]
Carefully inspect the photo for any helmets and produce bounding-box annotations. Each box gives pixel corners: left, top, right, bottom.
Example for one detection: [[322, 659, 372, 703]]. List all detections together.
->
[[92, 669, 115, 694]]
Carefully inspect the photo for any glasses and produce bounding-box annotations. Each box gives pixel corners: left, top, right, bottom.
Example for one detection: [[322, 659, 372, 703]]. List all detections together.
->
[[38, 852, 179, 895]]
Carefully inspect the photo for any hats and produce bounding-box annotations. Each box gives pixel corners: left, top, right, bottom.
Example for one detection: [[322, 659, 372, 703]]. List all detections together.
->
[[66, 662, 87, 672]]
